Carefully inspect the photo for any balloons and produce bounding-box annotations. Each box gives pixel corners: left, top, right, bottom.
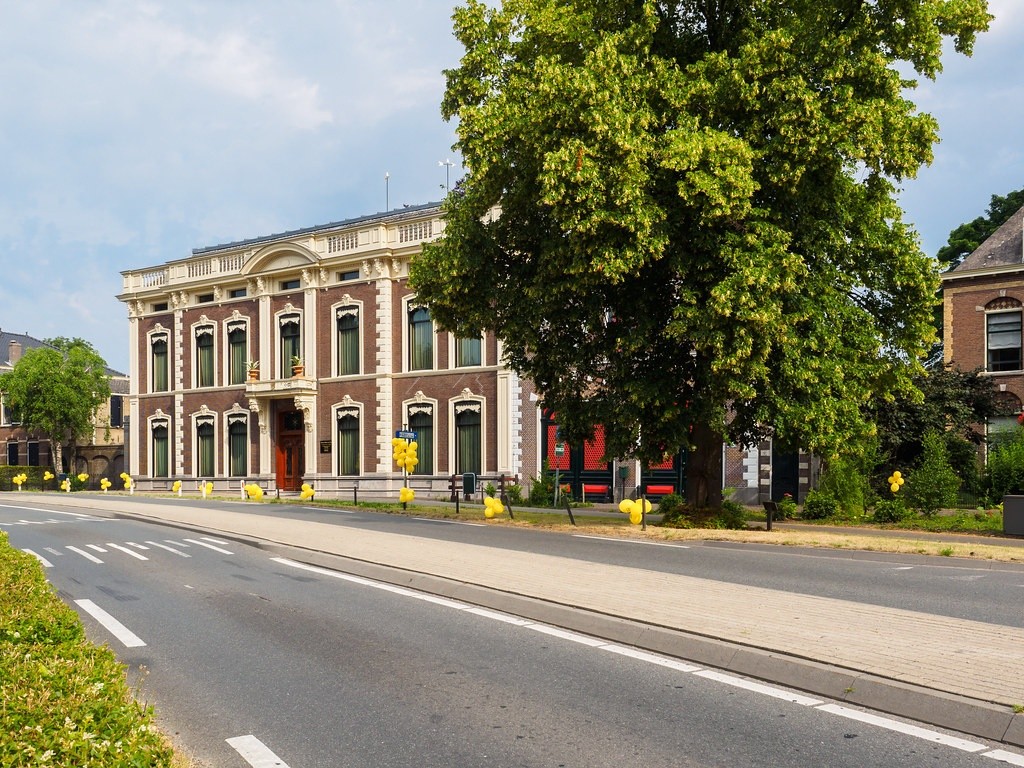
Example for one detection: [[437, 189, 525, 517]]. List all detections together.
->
[[300, 484, 315, 499], [888, 471, 904, 492], [43, 471, 54, 480], [484, 497, 505, 518], [400, 487, 415, 502], [246, 484, 262, 498], [619, 498, 652, 524], [78, 474, 89, 481], [100, 479, 112, 489], [12, 473, 28, 485], [198, 483, 213, 494], [61, 480, 71, 489], [392, 438, 418, 473], [120, 472, 135, 489], [172, 481, 181, 493]]
